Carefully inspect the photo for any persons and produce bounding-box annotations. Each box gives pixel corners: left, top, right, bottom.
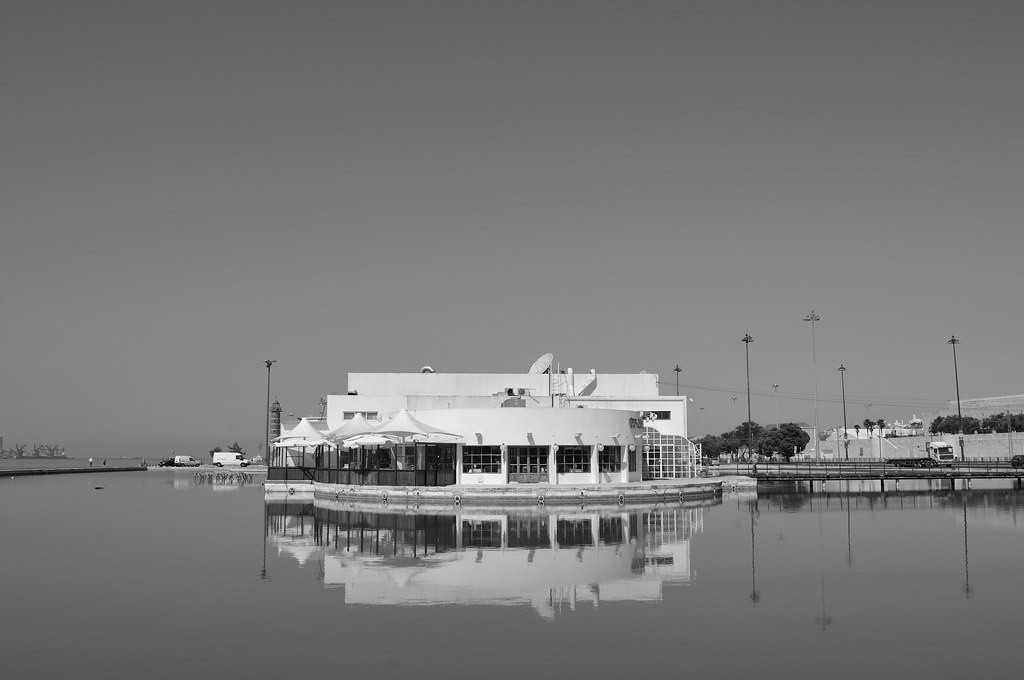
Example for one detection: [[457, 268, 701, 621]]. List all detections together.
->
[[89, 457, 93, 467]]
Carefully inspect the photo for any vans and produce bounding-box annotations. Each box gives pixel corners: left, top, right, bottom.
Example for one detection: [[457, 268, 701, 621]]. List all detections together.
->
[[212, 452, 250, 467], [173, 456, 200, 467]]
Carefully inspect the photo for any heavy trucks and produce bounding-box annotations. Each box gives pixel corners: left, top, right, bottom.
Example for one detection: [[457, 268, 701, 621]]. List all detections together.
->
[[883, 441, 956, 468]]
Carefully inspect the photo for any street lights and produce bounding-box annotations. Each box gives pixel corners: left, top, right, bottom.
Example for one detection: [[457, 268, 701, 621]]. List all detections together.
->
[[800, 310, 822, 461], [844, 494, 857, 574], [816, 493, 833, 631], [741, 333, 755, 460], [262, 359, 277, 464], [837, 364, 849, 462], [946, 335, 965, 461], [673, 364, 682, 397], [748, 498, 762, 605], [961, 477, 974, 596]]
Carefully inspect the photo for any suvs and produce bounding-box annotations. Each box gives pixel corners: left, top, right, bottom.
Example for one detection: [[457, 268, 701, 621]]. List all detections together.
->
[[1011, 454, 1024, 470], [703, 457, 721, 466]]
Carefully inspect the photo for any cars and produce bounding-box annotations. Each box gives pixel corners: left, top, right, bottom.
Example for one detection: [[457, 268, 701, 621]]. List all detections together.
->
[[158, 457, 182, 467]]
